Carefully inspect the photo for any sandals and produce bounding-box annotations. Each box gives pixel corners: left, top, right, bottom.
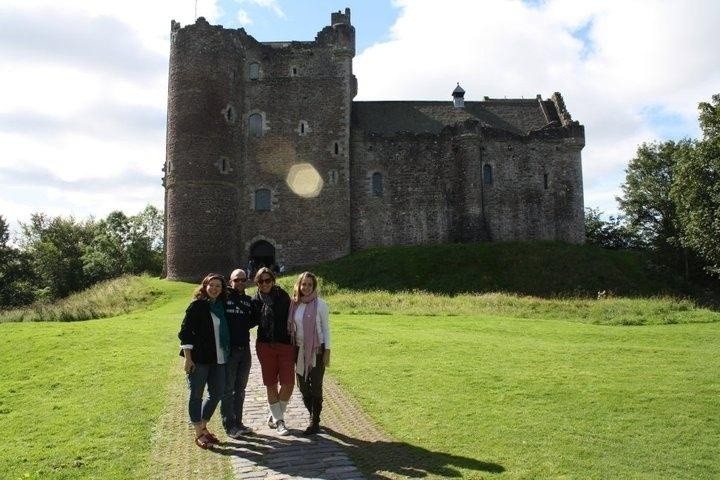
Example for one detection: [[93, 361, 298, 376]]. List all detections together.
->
[[195, 427, 218, 449]]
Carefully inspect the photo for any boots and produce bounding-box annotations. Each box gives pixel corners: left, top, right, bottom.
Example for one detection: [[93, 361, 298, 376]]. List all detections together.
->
[[302, 398, 324, 436]]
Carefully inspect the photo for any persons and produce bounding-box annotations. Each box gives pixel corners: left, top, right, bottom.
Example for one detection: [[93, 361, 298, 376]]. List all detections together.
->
[[220, 268, 254, 439], [177, 271, 232, 449], [248, 266, 298, 437], [286, 269, 333, 438]]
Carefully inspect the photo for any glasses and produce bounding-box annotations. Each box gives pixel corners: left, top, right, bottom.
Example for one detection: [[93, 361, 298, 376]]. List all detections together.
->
[[258, 278, 271, 283], [234, 278, 247, 282]]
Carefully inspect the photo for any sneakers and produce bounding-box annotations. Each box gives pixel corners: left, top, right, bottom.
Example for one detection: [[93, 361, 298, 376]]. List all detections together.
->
[[228, 423, 253, 439], [268, 415, 289, 435]]
